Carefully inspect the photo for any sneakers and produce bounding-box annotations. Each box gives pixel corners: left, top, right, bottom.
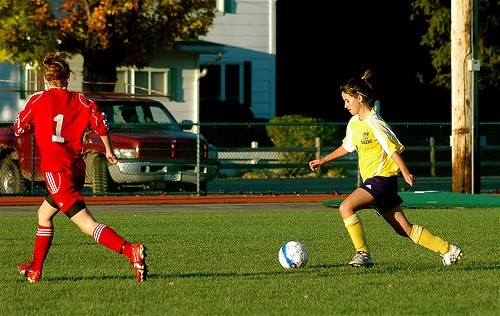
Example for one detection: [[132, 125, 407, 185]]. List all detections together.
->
[[441, 245, 463, 265], [130, 244, 147, 282], [18, 258, 42, 282], [348, 253, 373, 267]]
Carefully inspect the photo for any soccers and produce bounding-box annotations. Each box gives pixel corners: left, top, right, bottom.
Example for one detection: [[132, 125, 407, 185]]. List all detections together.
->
[[278, 240, 308, 268]]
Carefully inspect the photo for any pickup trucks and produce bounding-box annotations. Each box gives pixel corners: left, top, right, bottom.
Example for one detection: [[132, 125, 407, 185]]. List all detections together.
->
[[0, 92, 221, 196]]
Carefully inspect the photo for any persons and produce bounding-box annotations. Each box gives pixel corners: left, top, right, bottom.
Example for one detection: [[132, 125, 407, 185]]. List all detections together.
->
[[309, 69, 462, 267], [14, 54, 147, 282]]
